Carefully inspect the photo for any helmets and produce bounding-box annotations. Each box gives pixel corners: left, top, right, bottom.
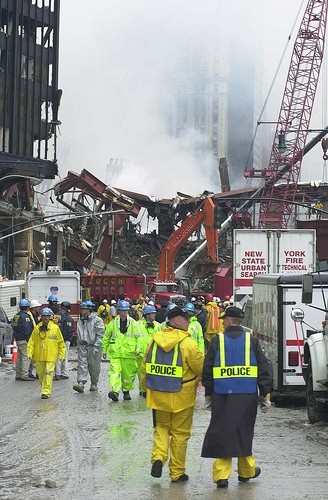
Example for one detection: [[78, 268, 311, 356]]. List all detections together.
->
[[103, 292, 236, 309], [29, 300, 42, 308], [143, 305, 157, 314], [116, 300, 130, 310], [47, 295, 59, 302], [60, 301, 71, 309], [80, 301, 95, 309], [19, 299, 30, 306], [41, 307, 53, 316]]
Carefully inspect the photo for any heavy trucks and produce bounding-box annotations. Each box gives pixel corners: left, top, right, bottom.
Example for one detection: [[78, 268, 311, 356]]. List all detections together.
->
[[81, 274, 187, 311]]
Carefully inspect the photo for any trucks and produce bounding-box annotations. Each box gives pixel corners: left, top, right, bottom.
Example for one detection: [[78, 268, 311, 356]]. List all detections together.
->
[[242, 270, 328, 393], [27, 267, 81, 346]]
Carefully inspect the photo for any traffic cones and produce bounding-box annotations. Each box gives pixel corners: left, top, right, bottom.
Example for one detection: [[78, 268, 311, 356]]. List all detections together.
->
[[11, 338, 18, 364]]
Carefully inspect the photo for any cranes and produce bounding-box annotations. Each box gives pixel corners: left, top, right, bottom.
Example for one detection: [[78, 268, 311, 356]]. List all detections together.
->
[[242, 0, 328, 229]]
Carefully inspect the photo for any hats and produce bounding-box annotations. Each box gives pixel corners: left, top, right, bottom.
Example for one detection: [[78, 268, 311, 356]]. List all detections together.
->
[[218, 306, 244, 319], [167, 307, 193, 318]]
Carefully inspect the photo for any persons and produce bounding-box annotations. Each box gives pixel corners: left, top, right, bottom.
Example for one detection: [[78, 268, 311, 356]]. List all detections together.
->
[[73, 301, 104, 392], [136, 305, 160, 397], [27, 299, 39, 379], [26, 310, 67, 399], [156, 304, 197, 343], [194, 304, 205, 334], [40, 295, 67, 380], [219, 296, 230, 332], [102, 301, 142, 402], [204, 294, 220, 342], [59, 301, 74, 379], [183, 303, 203, 380], [98, 296, 155, 330], [13, 299, 34, 381], [141, 308, 205, 481], [201, 306, 271, 487]]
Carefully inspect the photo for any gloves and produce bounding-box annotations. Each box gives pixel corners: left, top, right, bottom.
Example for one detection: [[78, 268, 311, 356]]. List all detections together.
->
[[140, 392, 147, 398], [260, 399, 271, 414], [204, 400, 212, 412]]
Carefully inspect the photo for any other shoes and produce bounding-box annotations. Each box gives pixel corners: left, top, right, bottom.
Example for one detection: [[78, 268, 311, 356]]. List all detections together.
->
[[15, 376, 35, 381], [42, 394, 48, 399], [55, 375, 69, 380], [73, 384, 84, 393], [217, 479, 228, 488], [171, 473, 189, 482], [123, 391, 131, 400], [108, 392, 119, 402], [90, 384, 98, 391], [238, 467, 261, 483], [151, 460, 163, 478]]
